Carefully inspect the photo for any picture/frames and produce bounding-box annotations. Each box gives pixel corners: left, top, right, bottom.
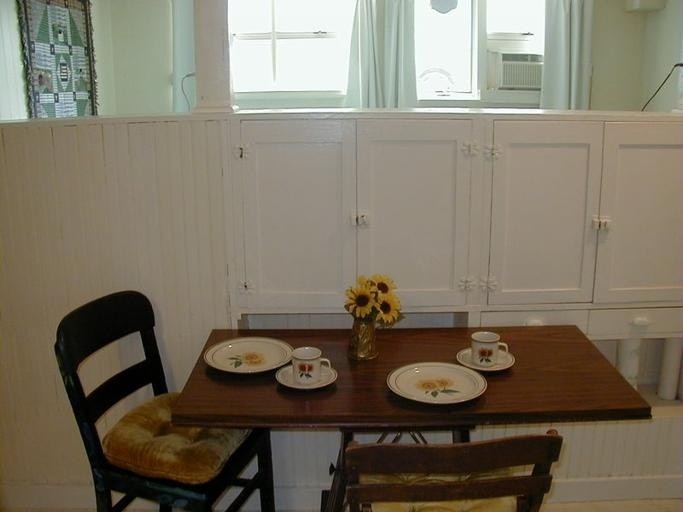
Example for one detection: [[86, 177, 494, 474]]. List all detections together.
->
[[15, 0, 101, 119]]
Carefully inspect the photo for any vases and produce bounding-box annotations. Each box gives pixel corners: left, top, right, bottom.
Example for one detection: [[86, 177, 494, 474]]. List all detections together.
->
[[348, 320, 380, 362]]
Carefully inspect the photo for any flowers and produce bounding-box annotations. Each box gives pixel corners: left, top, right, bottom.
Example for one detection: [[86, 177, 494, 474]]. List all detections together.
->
[[343, 271, 403, 326]]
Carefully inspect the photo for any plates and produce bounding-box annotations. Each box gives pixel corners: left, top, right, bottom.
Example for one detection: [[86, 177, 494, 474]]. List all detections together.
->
[[275, 364, 337, 393], [203, 335, 293, 373], [387, 360, 487, 406], [457, 348, 516, 373]]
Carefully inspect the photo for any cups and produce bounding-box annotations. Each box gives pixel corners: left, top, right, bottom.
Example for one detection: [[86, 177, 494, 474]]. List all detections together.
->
[[468, 331, 508, 365], [289, 347, 331, 384]]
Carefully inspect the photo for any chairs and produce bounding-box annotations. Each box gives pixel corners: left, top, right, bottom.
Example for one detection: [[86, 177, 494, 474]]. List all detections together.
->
[[318, 427, 568, 512], [54, 288, 279, 512]]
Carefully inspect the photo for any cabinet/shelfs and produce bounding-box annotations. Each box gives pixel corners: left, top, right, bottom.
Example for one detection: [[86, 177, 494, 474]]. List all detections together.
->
[[487, 118, 683, 309], [232, 113, 481, 312]]
